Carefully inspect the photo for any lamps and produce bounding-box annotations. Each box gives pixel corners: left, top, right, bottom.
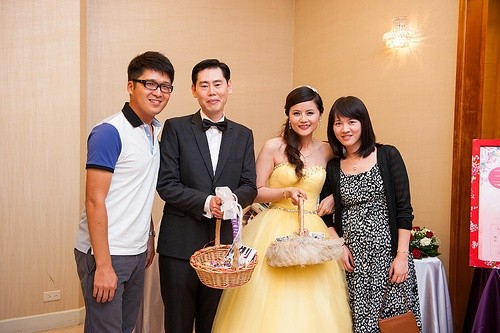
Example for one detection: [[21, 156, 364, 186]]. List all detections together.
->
[[383, 16, 414, 49]]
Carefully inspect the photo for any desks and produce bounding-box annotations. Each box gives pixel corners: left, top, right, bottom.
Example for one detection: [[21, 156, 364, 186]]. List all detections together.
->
[[413, 257, 453, 333]]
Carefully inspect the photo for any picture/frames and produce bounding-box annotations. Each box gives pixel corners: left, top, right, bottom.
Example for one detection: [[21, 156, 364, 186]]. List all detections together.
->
[[469, 138, 500, 270]]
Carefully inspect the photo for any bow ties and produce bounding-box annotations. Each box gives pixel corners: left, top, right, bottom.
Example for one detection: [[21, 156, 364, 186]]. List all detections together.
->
[[202, 118, 228, 133]]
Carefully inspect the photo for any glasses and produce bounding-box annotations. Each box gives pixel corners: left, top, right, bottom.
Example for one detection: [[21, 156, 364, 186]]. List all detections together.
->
[[131, 79, 174, 94]]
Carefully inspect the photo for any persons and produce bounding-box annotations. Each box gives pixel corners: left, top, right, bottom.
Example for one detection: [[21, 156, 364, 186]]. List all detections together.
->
[[319, 96, 423, 333], [74, 52, 175, 333], [210, 87, 355, 333], [156, 59, 258, 333]]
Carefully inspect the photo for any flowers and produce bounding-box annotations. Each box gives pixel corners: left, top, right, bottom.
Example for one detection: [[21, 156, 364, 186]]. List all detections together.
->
[[409, 225, 442, 260]]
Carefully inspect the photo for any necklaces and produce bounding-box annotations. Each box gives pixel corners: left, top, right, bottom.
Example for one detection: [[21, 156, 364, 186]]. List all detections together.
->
[[349, 155, 361, 170], [300, 147, 313, 164]]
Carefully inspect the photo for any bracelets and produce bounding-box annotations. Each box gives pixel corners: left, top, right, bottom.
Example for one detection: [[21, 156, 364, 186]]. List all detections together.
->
[[282, 188, 288, 200], [149, 231, 155, 237], [396, 249, 410, 253]]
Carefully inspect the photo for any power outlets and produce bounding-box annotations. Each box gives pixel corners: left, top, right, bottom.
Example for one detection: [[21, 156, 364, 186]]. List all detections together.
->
[[44, 290, 60, 301]]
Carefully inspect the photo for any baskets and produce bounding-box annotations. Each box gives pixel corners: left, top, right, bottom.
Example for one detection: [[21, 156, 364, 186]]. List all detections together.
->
[[264, 194, 345, 267], [189, 214, 260, 290]]
